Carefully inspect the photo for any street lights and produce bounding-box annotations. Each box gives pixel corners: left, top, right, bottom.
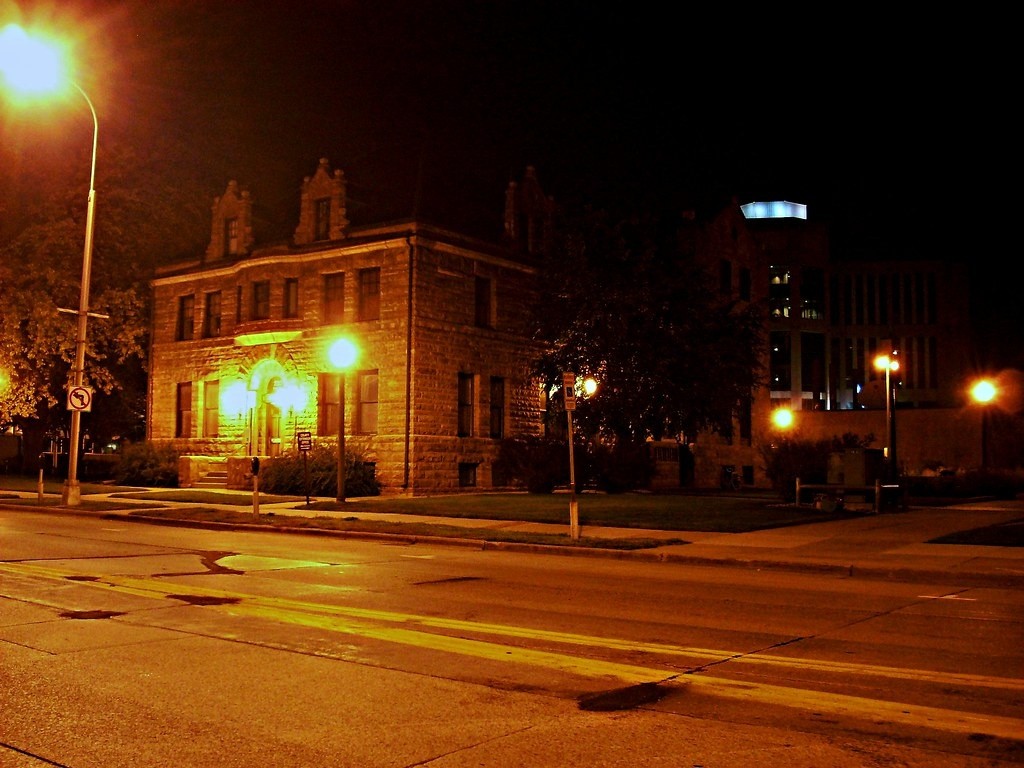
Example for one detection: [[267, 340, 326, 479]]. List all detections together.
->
[[332, 338, 358, 504], [5, 37, 102, 505], [974, 381, 993, 470], [875, 356, 892, 467]]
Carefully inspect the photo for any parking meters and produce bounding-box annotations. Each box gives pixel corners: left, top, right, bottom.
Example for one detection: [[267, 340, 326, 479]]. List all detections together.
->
[[250, 456, 260, 521]]
